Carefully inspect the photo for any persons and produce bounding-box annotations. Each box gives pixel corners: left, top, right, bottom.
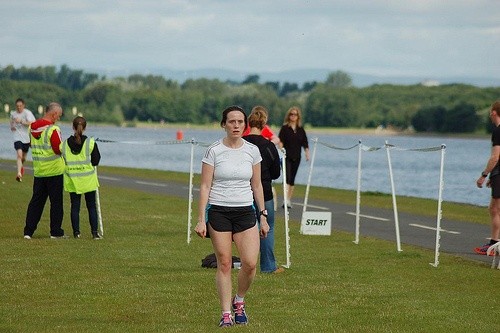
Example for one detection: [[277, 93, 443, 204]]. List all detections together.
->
[[63, 116, 103, 239], [278, 106, 309, 208], [194, 106, 269, 326], [473, 102, 500, 256], [242, 106, 280, 144], [23, 102, 69, 239], [241, 110, 284, 274], [9, 99, 37, 182]]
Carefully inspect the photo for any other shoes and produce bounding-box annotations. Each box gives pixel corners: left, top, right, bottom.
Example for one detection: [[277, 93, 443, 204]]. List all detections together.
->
[[16, 175, 22, 181], [21, 167, 24, 174], [51, 229, 64, 238], [24, 233, 31, 238], [274, 267, 284, 273], [93, 233, 103, 239], [74, 231, 81, 238]]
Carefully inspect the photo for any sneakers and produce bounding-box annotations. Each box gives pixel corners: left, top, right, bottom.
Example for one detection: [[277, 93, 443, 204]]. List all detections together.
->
[[218, 312, 234, 327], [232, 295, 248, 324], [474, 244, 499, 255]]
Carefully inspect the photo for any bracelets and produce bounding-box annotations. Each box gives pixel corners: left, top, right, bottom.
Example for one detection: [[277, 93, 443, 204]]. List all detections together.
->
[[482, 172, 487, 177], [260, 210, 268, 216], [20, 121, 22, 124]]
